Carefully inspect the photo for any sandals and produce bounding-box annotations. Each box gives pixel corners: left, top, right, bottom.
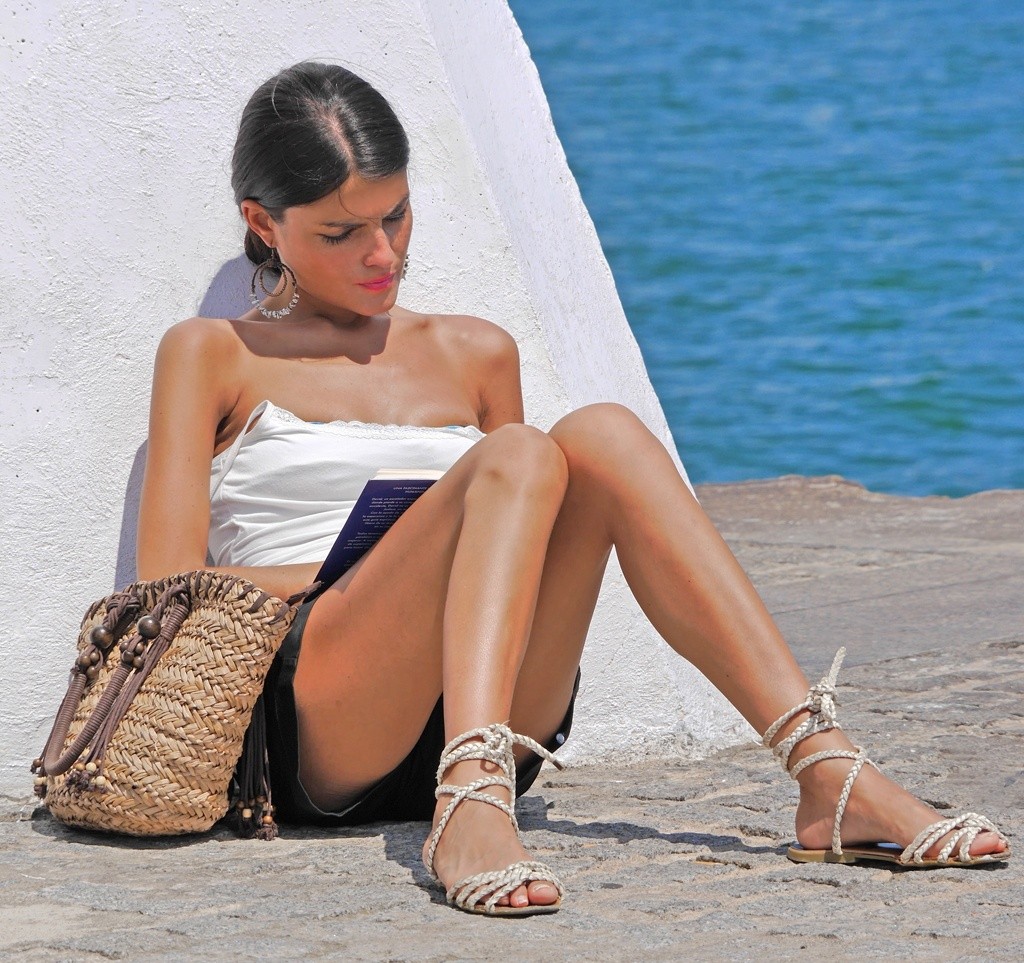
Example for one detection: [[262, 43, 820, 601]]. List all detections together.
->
[[758, 644, 1010, 869], [423, 731, 562, 917]]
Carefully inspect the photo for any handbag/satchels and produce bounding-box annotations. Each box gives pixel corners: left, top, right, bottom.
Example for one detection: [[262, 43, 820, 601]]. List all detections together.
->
[[33, 568, 323, 840]]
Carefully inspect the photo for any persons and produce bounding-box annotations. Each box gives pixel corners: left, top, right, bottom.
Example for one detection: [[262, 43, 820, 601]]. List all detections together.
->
[[135, 62, 1014, 917]]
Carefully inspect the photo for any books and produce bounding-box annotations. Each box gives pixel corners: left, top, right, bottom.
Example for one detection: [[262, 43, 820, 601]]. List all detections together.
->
[[312, 465, 446, 585]]
